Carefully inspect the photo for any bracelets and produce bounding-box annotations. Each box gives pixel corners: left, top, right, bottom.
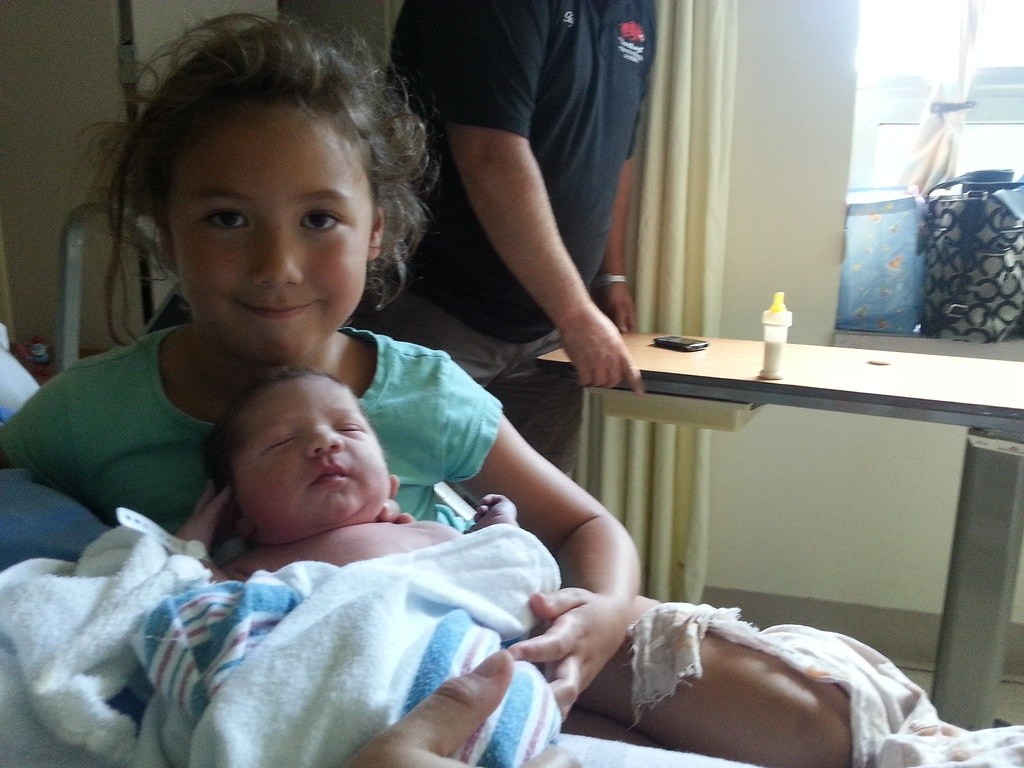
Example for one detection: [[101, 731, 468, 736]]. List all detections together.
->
[[590, 273, 628, 289], [113, 505, 216, 565]]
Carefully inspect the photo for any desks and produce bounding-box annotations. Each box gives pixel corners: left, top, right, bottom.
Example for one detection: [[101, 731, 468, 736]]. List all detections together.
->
[[536, 332, 1024, 731]]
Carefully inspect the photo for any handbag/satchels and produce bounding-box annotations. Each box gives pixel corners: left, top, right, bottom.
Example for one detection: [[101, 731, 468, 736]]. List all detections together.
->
[[836, 183, 926, 334], [915, 166, 1024, 344]]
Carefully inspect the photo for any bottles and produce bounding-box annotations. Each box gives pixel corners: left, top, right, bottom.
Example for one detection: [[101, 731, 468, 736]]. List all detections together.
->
[[757, 291, 793, 380]]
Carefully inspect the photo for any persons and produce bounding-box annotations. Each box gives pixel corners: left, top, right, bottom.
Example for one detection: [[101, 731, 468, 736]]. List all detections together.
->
[[168, 359, 563, 768], [362, 2, 659, 488], [1, 14, 860, 768]]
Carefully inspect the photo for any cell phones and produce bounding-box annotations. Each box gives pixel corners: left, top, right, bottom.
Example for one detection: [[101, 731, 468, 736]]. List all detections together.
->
[[654, 336, 708, 352]]
